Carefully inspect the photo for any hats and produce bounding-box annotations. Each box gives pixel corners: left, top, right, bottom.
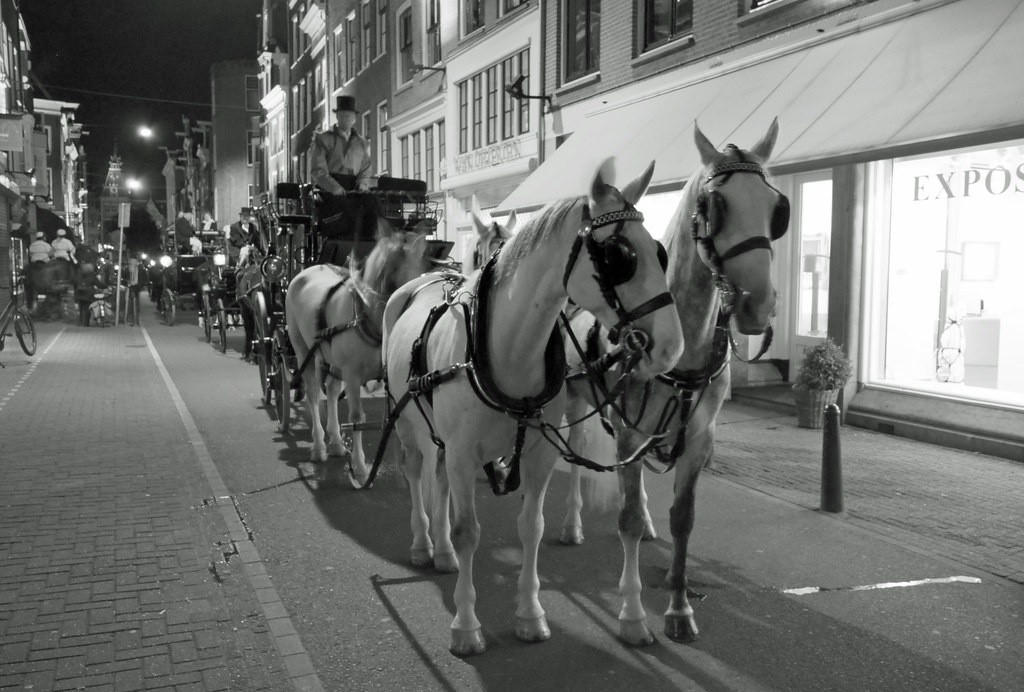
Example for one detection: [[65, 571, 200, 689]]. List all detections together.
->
[[332, 96, 360, 114], [184, 207, 192, 213], [56, 229, 66, 236], [36, 231, 43, 238], [239, 207, 253, 216]]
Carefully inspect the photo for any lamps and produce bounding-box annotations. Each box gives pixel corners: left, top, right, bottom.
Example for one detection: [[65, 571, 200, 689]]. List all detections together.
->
[[409, 64, 444, 72], [507, 74, 553, 103]]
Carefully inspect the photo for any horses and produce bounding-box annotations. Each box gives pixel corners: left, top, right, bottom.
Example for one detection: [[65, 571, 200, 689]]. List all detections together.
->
[[379, 155, 687, 658], [471, 207, 517, 285], [285, 216, 433, 490], [553, 115, 789, 649]]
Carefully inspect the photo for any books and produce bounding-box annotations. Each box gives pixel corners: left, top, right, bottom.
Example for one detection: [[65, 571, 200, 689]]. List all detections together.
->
[[491, 1, 1024, 217]]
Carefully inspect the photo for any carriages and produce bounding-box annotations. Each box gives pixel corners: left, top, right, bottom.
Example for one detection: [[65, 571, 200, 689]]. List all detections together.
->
[[252, 118, 789, 660], [20, 223, 275, 356]]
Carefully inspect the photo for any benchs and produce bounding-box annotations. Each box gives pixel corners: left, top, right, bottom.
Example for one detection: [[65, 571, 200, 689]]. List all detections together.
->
[[272, 181, 309, 226], [312, 173, 439, 230], [168, 230, 227, 254]]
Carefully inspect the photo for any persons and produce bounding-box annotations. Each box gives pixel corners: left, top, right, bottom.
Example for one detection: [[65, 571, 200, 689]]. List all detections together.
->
[[310, 96, 372, 196], [75, 264, 108, 324], [123, 255, 143, 326], [176, 207, 202, 254], [52, 228, 74, 261], [229, 207, 260, 264], [200, 211, 217, 230], [29, 232, 52, 263]]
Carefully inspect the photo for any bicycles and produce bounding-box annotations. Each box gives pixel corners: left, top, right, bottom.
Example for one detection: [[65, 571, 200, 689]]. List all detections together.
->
[[0, 278, 38, 370]]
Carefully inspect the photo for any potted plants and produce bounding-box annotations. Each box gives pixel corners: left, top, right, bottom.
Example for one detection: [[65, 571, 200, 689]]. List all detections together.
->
[[790, 338, 852, 429]]
